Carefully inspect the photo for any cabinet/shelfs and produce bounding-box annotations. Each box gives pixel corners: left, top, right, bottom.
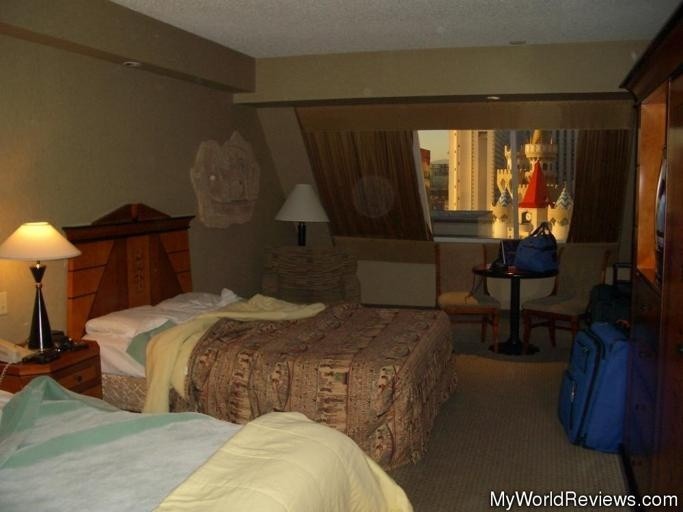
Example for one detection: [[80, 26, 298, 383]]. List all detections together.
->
[[617, 73, 683, 512]]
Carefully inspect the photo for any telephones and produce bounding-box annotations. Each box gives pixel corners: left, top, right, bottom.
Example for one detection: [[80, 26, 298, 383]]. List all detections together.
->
[[0, 338, 35, 364]]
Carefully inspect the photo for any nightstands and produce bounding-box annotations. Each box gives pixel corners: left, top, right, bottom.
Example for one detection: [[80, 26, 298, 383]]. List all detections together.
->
[[0, 339, 101, 399]]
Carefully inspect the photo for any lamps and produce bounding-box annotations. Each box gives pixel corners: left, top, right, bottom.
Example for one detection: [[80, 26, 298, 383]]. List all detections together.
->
[[0, 220, 82, 364], [274, 184, 330, 246]]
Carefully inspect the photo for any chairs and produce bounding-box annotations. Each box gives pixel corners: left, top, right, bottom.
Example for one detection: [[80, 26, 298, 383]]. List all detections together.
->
[[260, 245, 362, 307], [433, 242, 499, 354], [520, 246, 608, 355]]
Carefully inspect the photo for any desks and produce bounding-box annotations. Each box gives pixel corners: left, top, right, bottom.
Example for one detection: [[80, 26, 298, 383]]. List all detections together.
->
[[470, 262, 558, 355]]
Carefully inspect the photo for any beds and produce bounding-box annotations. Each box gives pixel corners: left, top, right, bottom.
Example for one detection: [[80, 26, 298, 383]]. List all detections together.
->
[[61, 202, 450, 469], [0, 389, 358, 511]]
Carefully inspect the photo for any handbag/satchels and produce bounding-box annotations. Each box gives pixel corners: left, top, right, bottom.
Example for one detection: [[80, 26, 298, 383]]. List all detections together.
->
[[513, 221, 558, 272], [498, 239, 521, 266], [584, 282, 631, 323]]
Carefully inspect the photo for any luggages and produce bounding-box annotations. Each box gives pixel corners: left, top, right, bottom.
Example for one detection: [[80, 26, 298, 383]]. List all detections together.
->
[[556, 321, 630, 454]]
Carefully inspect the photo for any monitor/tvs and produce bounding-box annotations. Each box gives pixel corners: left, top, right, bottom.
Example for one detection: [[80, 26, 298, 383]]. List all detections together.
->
[[652, 159, 665, 286]]
